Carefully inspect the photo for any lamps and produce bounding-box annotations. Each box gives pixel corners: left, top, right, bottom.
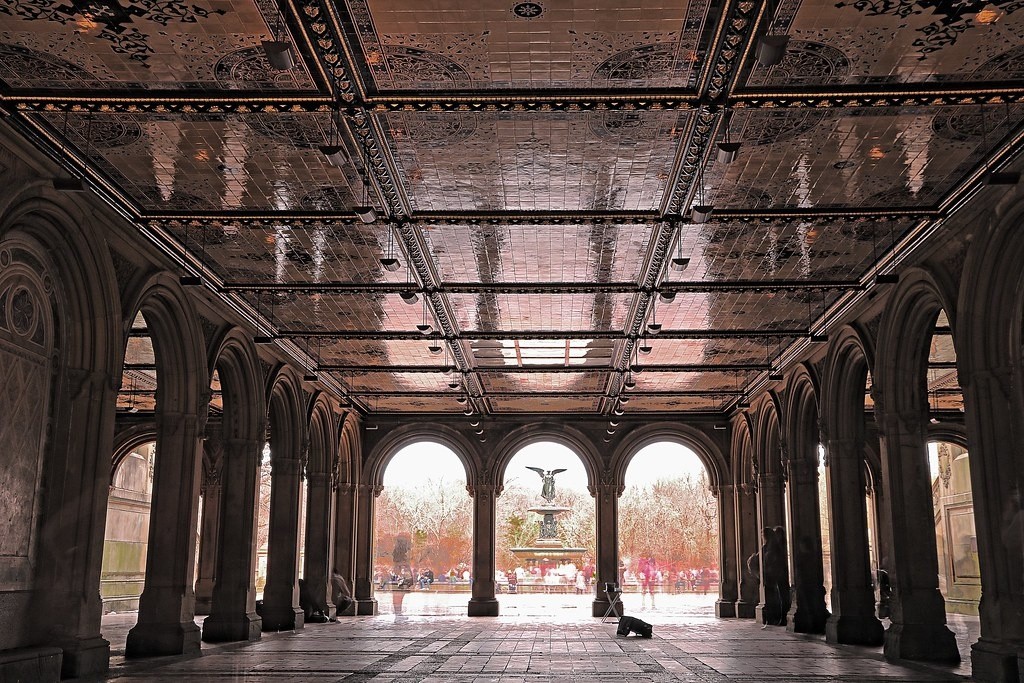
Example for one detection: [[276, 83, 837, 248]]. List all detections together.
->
[[691, 157, 715, 222], [670, 221, 690, 271], [660, 256, 677, 304], [714, 105, 742, 163], [337, 290, 783, 434], [318, 68, 350, 168], [52, 113, 93, 191], [872, 216, 899, 284], [755, 0, 791, 66], [260, 1, 299, 71], [303, 337, 320, 382], [380, 225, 401, 272], [398, 250, 419, 305], [351, 138, 378, 224], [179, 225, 207, 287], [808, 288, 827, 343], [252, 293, 275, 344], [981, 101, 1021, 185]]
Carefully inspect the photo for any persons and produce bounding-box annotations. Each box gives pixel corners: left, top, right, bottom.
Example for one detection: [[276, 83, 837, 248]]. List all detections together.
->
[[772, 526, 789, 566], [331, 568, 352, 615], [495, 558, 597, 596], [793, 534, 833, 633], [374, 564, 472, 591], [762, 526, 791, 625], [619, 554, 719, 614]]
